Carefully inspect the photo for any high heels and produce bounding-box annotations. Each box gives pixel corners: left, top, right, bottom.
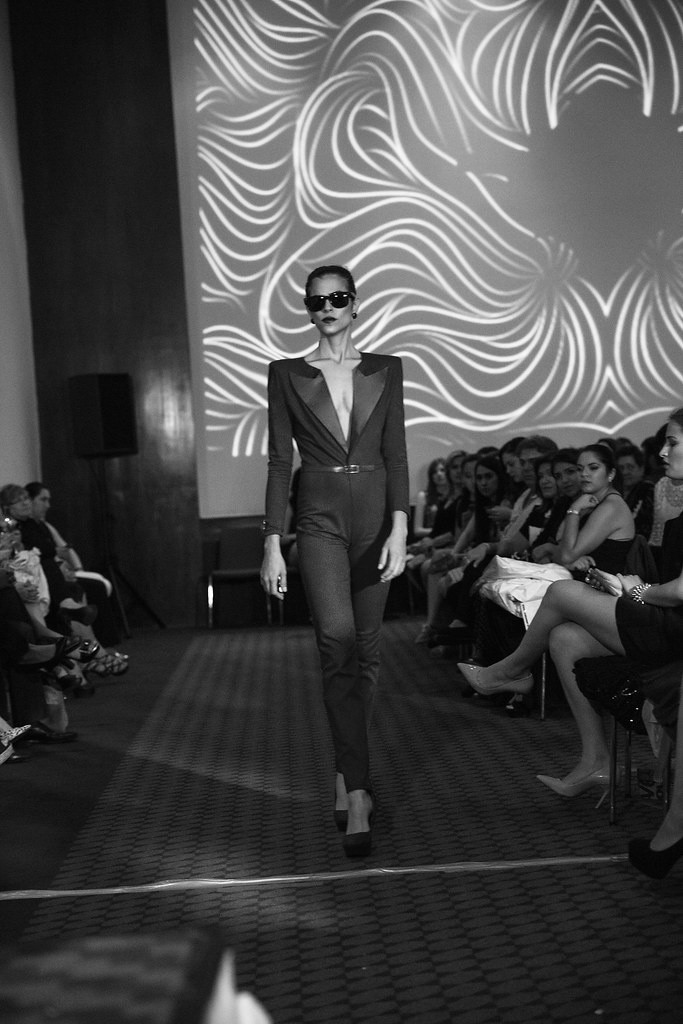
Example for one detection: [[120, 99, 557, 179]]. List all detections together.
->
[[36, 632, 130, 699], [628, 835, 683, 879], [343, 787, 378, 858], [457, 663, 534, 704], [15, 637, 66, 685], [333, 788, 348, 831], [537, 760, 620, 810], [58, 603, 97, 631]]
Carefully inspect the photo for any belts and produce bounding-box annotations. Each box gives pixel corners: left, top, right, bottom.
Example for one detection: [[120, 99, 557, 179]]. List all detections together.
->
[[300, 463, 386, 473]]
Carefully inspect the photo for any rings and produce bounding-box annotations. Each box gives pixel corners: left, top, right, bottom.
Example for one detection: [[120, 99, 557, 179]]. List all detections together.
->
[[464, 556, 470, 564]]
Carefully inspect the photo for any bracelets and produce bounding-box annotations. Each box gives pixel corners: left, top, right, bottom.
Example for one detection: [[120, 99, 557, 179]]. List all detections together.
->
[[630, 582, 652, 605], [566, 509, 581, 516], [482, 543, 491, 551]]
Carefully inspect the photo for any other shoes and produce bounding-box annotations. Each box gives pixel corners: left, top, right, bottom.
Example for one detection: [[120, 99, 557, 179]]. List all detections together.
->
[[429, 644, 452, 658], [4, 751, 32, 764], [414, 624, 442, 644], [23, 721, 76, 745], [438, 623, 472, 637], [0, 724, 31, 746]]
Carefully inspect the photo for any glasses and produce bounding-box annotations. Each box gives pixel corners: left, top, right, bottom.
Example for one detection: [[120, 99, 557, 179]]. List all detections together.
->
[[303, 291, 355, 311]]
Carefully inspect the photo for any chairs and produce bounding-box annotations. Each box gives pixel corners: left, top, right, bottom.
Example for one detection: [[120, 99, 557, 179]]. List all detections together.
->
[[277, 543, 299, 626], [204, 521, 275, 630]]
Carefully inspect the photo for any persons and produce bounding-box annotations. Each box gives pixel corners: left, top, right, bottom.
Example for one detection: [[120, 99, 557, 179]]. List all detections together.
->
[[0, 479, 128, 771], [410, 401, 682, 880], [260, 264, 410, 860]]
